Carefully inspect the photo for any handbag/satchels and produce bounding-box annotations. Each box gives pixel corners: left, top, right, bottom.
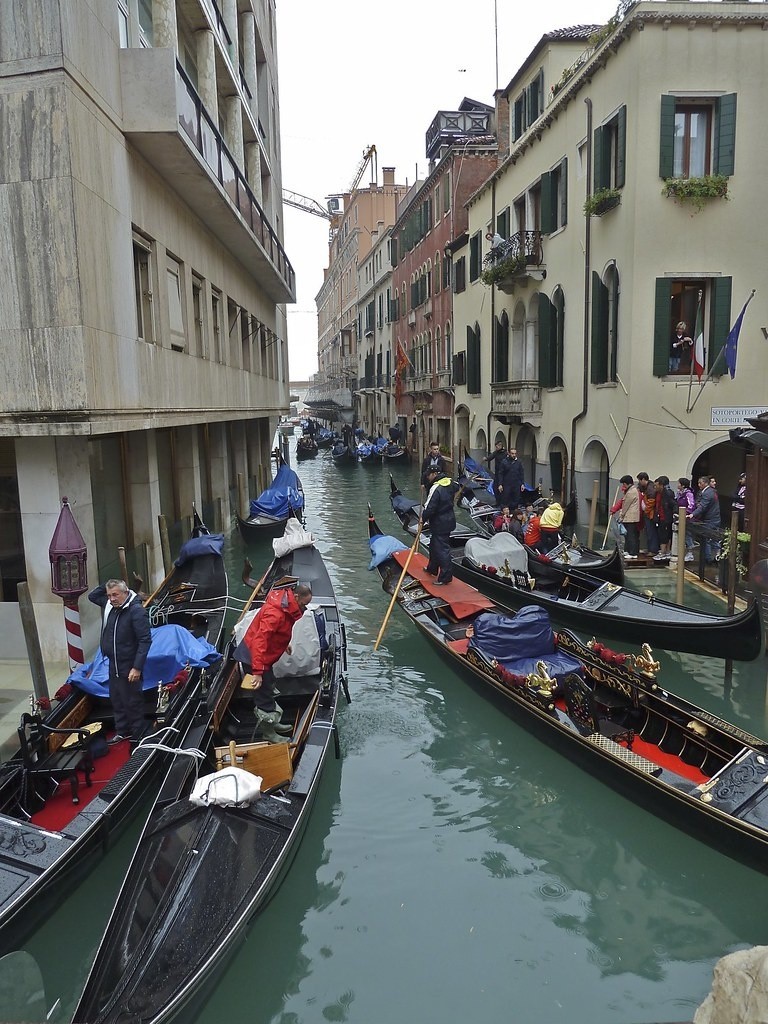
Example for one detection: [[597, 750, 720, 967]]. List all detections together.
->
[[83, 736, 108, 758], [188, 766, 263, 810], [618, 523, 628, 535]]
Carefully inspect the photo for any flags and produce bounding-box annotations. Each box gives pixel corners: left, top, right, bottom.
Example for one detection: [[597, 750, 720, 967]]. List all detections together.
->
[[393, 341, 408, 404], [724, 304, 746, 380], [695, 304, 703, 384]]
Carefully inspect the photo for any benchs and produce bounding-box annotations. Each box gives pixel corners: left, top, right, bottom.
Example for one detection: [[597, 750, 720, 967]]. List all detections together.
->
[[475, 605, 586, 699]]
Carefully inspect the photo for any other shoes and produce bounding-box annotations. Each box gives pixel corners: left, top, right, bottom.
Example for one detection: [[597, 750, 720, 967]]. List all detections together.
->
[[129, 740, 139, 756], [107, 733, 132, 746], [423, 566, 437, 577], [432, 580, 451, 585], [684, 551, 694, 562], [706, 557, 713, 564]]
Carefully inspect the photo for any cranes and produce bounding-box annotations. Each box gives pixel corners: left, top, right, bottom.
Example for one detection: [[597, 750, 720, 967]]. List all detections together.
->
[[282, 144, 378, 242]]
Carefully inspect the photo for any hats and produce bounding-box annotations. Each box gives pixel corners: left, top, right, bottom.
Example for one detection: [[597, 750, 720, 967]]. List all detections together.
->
[[423, 464, 440, 475], [620, 475, 634, 484]]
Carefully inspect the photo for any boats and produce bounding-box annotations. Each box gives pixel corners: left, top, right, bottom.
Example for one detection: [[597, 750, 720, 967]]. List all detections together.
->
[[0, 500, 231, 961], [235, 447, 305, 534], [296, 417, 408, 463], [462, 445, 543, 506], [458, 470, 624, 585], [366, 501, 768, 861], [66, 517, 352, 1023], [389, 472, 764, 661]]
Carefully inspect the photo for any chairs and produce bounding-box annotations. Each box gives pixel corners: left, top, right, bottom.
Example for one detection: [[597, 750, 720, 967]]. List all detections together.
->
[[512, 568, 531, 594], [561, 673, 633, 753], [17, 712, 93, 803]]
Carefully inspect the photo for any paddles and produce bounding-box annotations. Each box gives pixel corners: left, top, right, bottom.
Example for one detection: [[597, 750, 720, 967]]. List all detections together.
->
[[231, 557, 276, 635], [454, 460, 484, 501], [322, 439, 339, 458], [143, 567, 176, 608], [416, 488, 424, 553], [374, 523, 424, 651]]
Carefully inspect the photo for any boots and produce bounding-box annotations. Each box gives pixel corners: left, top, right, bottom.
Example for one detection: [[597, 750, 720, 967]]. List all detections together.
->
[[253, 702, 293, 745]]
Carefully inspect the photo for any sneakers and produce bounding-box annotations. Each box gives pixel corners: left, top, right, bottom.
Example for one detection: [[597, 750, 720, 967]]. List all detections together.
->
[[622, 548, 672, 560]]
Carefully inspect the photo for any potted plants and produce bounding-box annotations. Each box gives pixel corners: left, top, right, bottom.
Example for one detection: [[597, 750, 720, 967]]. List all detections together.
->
[[662, 174, 732, 218], [582, 187, 622, 218], [482, 256, 537, 288]]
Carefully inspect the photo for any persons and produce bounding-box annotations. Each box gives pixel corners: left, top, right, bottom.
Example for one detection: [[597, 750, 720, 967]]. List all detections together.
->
[[389, 423, 402, 443], [420, 442, 448, 492], [485, 233, 505, 249], [88, 578, 153, 745], [232, 584, 313, 745], [421, 465, 458, 584], [610, 472, 746, 562], [306, 417, 321, 438], [482, 441, 525, 506], [669, 321, 693, 372], [341, 419, 362, 445], [494, 501, 569, 547]]
